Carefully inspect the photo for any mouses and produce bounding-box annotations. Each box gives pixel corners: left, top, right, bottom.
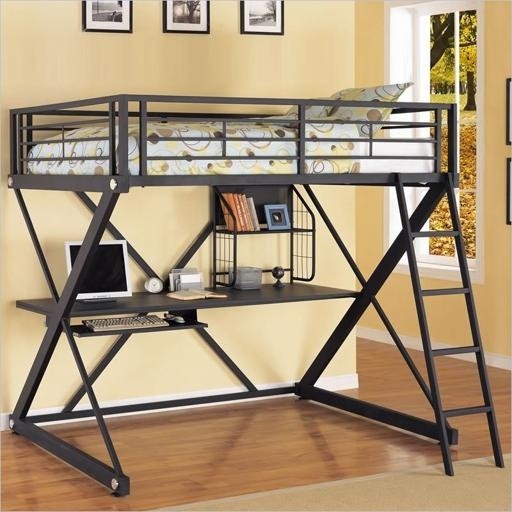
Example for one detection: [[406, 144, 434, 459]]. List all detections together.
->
[[167, 314, 185, 323]]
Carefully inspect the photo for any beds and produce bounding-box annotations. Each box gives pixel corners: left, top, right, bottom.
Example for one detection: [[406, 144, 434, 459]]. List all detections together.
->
[[7, 94, 506, 498]]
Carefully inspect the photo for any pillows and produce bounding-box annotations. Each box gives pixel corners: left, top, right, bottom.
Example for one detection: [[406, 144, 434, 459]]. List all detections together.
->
[[286, 81, 415, 138]]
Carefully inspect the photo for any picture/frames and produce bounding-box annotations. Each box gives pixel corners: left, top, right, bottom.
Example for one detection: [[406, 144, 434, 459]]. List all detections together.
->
[[163, 0, 210, 34], [506, 158, 512, 224], [506, 78, 512, 145], [81, 0, 133, 34], [240, 0, 284, 35]]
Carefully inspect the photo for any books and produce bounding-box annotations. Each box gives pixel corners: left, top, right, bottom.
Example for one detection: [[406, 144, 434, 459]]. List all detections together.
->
[[220, 191, 261, 231], [167, 288, 228, 300]]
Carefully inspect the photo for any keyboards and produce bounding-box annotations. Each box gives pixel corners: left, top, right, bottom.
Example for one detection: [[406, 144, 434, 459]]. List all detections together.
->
[[82, 315, 170, 332]]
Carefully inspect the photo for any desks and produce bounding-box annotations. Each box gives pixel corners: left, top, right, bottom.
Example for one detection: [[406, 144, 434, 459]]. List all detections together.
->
[[8, 283, 459, 497]]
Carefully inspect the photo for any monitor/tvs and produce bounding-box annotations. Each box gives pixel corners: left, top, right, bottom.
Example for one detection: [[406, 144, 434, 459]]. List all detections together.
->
[[65, 240, 132, 303]]
